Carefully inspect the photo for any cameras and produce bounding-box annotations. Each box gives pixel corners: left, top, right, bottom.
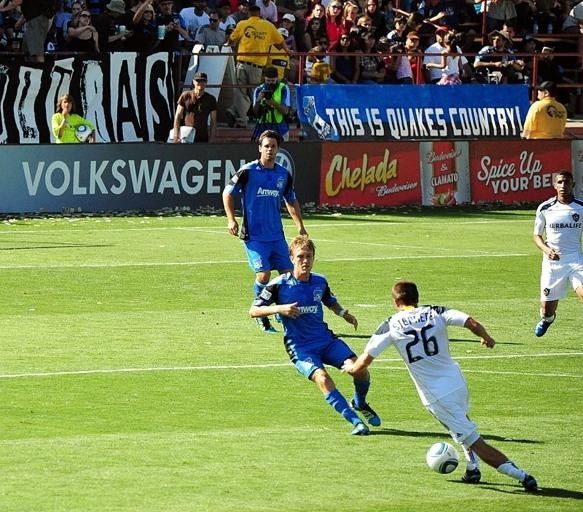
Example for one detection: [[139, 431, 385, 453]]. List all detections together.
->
[[261, 83, 273, 101]]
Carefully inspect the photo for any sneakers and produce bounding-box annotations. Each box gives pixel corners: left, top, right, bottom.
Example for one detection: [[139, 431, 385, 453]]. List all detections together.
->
[[460, 467, 482, 483], [351, 424, 370, 436], [255, 317, 278, 333], [520, 473, 538, 493], [535, 312, 557, 337], [350, 398, 381, 427]]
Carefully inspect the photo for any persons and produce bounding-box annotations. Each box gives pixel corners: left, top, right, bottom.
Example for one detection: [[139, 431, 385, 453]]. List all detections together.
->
[[225, 24, 238, 50], [133, 0, 161, 53], [275, 0, 308, 44], [343, 2, 358, 32], [86, 0, 104, 16], [305, 4, 326, 32], [310, 46, 330, 83], [157, 0, 189, 41], [535, 0, 562, 36], [350, 27, 403, 54], [380, 0, 395, 30], [194, 10, 225, 53], [174, 73, 217, 144], [389, 44, 414, 84], [521, 36, 537, 53], [280, 13, 300, 52], [325, 0, 344, 52], [534, 171, 583, 337], [15, 0, 56, 63], [418, 0, 460, 27], [250, 236, 381, 435], [52, 94, 96, 144], [255, 0, 278, 29], [426, 30, 463, 86], [229, 0, 256, 24], [561, 2, 583, 33], [502, 22, 514, 39], [266, 28, 290, 82], [304, 18, 321, 51], [305, 35, 335, 84], [364, 0, 385, 22], [404, 31, 424, 84], [341, 282, 538, 493], [219, 0, 236, 31], [360, 32, 387, 85], [330, 33, 360, 84], [514, 0, 537, 34], [62, 0, 86, 43], [521, 81, 566, 140], [95, 0, 132, 51], [474, 30, 525, 84], [357, 16, 372, 32], [223, 130, 309, 334], [126, 0, 148, 30], [178, 0, 210, 41], [538, 47, 562, 81], [386, 16, 408, 45], [224, 5, 294, 128], [67, 10, 100, 57], [422, 26, 472, 84], [474, 0, 521, 30], [0, 0, 28, 52], [253, 67, 292, 142]]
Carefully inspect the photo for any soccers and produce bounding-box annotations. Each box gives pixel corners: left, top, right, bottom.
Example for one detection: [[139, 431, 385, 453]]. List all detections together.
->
[[75, 125, 92, 143], [425, 441, 460, 474]]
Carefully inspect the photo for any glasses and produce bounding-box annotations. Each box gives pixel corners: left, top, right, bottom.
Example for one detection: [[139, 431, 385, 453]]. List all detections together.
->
[[144, 10, 153, 14], [80, 13, 92, 19], [311, 21, 319, 25], [316, 41, 327, 45], [72, 7, 80, 10], [492, 37, 501, 41], [208, 18, 218, 23]]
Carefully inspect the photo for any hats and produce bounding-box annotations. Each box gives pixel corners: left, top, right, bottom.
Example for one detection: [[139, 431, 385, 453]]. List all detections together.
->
[[487, 28, 514, 50], [224, 23, 236, 31], [519, 34, 539, 44], [105, 0, 126, 14], [535, 80, 557, 94], [193, 72, 207, 81], [277, 27, 289, 38], [281, 13, 296, 22], [263, 66, 279, 79], [435, 25, 449, 33], [407, 31, 420, 39]]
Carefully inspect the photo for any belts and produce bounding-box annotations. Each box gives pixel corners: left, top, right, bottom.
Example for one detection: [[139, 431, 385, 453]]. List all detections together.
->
[[239, 60, 263, 69]]
